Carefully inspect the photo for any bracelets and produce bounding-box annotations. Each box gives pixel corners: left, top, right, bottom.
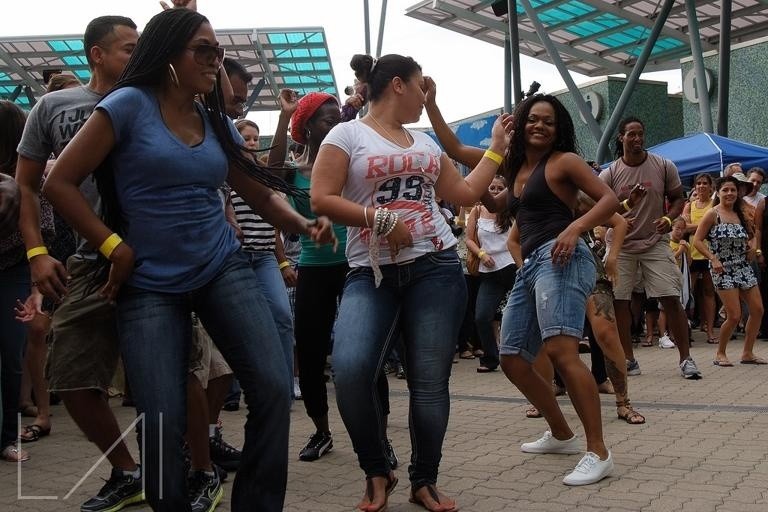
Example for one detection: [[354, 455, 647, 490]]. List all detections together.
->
[[754, 247, 763, 257], [30, 282, 41, 286], [623, 199, 633, 213], [362, 203, 371, 228], [455, 219, 466, 228], [26, 245, 49, 261], [98, 232, 123, 258], [476, 251, 486, 259], [369, 206, 398, 290], [278, 261, 291, 271], [662, 215, 672, 227], [755, 249, 764, 257], [275, 260, 295, 275]]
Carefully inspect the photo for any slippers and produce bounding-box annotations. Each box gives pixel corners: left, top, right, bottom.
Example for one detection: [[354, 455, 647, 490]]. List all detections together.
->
[[21, 406, 53, 418], [739, 356, 766, 365], [4, 446, 30, 465], [705, 335, 718, 343], [459, 355, 475, 360], [17, 423, 52, 445], [712, 358, 733, 367]]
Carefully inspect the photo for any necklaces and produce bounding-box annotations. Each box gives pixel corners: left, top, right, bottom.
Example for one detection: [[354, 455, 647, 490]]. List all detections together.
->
[[521, 182, 526, 191], [368, 111, 425, 173]]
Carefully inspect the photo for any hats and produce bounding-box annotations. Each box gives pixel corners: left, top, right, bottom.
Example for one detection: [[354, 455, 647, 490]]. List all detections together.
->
[[731, 173, 754, 196], [290, 93, 340, 144]]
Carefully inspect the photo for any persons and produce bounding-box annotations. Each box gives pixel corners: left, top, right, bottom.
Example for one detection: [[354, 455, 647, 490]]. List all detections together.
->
[[688, 188, 700, 203], [192, 59, 255, 477], [724, 163, 743, 177], [743, 167, 766, 208], [308, 52, 514, 512], [0, 99, 44, 464], [596, 120, 703, 380], [732, 173, 755, 228], [224, 122, 295, 416], [464, 174, 511, 374], [41, 5, 340, 512], [657, 216, 694, 351], [16, 74, 79, 443], [269, 89, 347, 461], [693, 175, 768, 367], [422, 74, 622, 486], [631, 293, 657, 347], [13, 0, 245, 512], [525, 190, 645, 427], [731, 172, 765, 342], [463, 174, 520, 374], [741, 167, 767, 342], [281, 145, 303, 401], [681, 174, 720, 345]]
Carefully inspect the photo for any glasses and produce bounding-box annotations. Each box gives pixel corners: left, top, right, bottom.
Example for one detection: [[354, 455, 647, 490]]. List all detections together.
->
[[234, 101, 249, 114], [182, 42, 225, 66], [673, 228, 685, 234]]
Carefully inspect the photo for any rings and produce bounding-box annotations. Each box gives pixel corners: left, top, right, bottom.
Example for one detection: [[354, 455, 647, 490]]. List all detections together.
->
[[556, 249, 568, 259], [559, 248, 568, 257]]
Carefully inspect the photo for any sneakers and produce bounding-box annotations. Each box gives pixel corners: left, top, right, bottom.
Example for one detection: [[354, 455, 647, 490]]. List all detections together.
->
[[293, 377, 301, 399], [550, 383, 566, 395], [384, 441, 397, 469], [598, 377, 614, 394], [299, 432, 332, 463], [80, 465, 145, 512], [657, 335, 676, 349], [677, 357, 702, 381], [624, 359, 638, 377], [182, 465, 224, 512], [520, 432, 581, 454], [395, 364, 405, 379], [562, 450, 613, 486], [208, 428, 245, 472], [382, 361, 391, 375]]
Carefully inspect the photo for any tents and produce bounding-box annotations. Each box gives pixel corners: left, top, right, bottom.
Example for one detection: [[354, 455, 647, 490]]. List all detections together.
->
[[593, 128, 768, 190]]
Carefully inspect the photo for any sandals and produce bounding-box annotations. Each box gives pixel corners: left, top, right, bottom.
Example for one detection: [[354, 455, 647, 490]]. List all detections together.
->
[[408, 480, 457, 512], [614, 399, 646, 425], [641, 334, 653, 347], [525, 407, 541, 417], [358, 467, 398, 511]]
